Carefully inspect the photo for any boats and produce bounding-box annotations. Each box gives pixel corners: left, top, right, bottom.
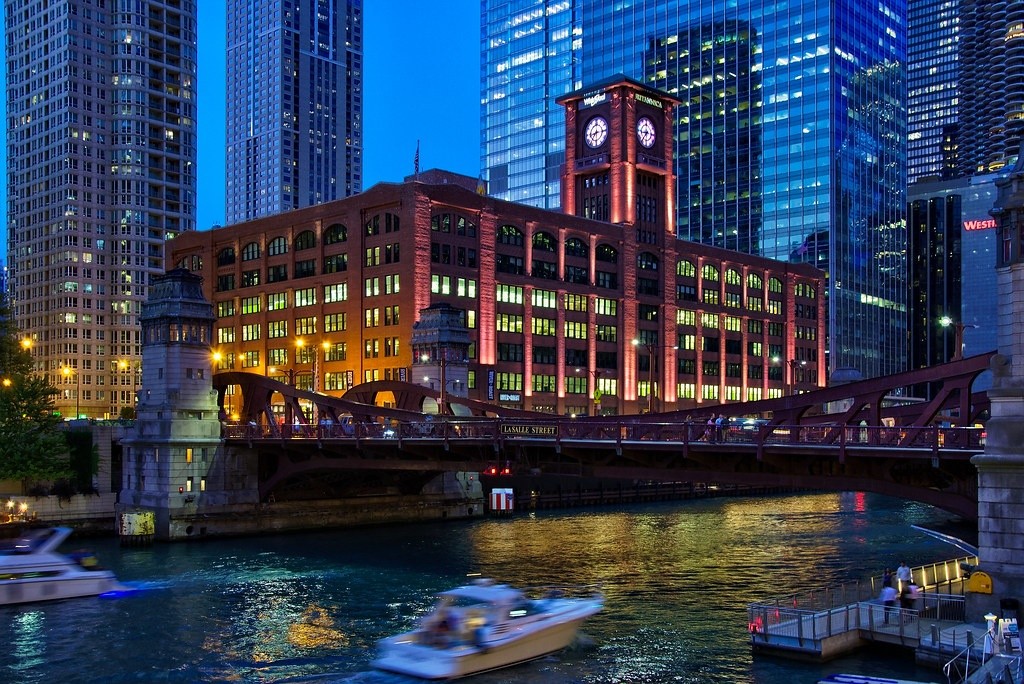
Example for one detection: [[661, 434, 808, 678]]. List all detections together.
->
[[0, 526, 119, 606], [367, 576, 605, 683]]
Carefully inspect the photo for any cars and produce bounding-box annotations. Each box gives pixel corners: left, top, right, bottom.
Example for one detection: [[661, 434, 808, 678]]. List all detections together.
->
[[343, 416, 382, 437], [725, 417, 772, 440]]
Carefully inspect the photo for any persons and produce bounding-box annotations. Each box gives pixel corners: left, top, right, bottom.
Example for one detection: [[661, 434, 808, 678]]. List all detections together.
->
[[881, 566, 896, 589], [700, 412, 729, 441], [896, 562, 912, 595], [899, 581, 918, 622], [882, 586, 897, 625]]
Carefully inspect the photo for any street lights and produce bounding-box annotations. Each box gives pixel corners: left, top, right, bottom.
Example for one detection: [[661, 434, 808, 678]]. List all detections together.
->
[[62, 368, 80, 420], [214, 351, 244, 419], [631, 339, 679, 414], [421, 348, 461, 415], [120, 360, 136, 419], [297, 339, 330, 424], [939, 316, 980, 360], [774, 357, 808, 397], [270, 368, 313, 386], [576, 368, 612, 416]]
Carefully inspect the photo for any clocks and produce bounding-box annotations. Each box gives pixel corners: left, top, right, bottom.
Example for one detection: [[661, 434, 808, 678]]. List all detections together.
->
[[636, 117, 657, 149], [584, 117, 609, 149]]
